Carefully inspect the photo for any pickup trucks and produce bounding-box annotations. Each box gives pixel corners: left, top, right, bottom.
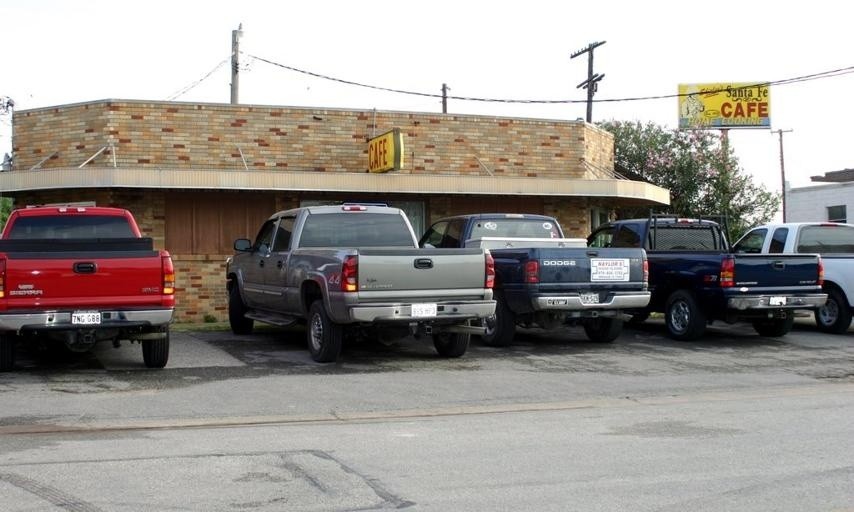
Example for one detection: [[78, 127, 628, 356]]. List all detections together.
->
[[419, 212, 652, 348], [730, 221, 854, 335], [586, 208, 828, 341], [2, 206, 176, 372], [225, 205, 498, 362]]
